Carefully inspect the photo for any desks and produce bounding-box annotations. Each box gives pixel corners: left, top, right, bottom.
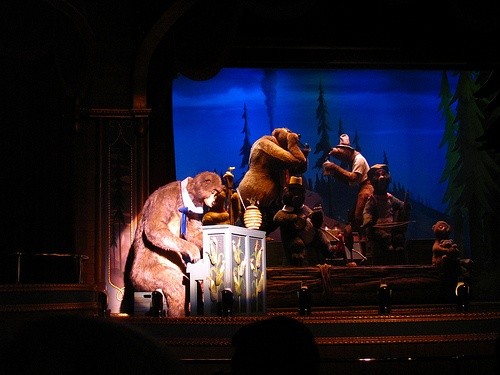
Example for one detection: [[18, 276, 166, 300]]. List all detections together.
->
[[190, 224, 267, 317], [16, 252, 88, 284]]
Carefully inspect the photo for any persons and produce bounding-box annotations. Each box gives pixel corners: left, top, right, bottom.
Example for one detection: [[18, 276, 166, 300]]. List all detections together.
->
[[323, 145, 374, 224], [231, 316, 322, 375], [0, 304, 189, 374]]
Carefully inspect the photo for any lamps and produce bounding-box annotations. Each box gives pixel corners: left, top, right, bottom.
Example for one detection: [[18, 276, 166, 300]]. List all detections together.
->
[[456, 281, 468, 313], [299, 285, 312, 316], [379, 284, 392, 315], [152, 291, 163, 318], [222, 290, 235, 317]]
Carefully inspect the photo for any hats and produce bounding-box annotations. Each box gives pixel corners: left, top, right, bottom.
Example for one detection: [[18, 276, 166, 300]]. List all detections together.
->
[[335, 133, 355, 151], [282, 176, 306, 191]]
